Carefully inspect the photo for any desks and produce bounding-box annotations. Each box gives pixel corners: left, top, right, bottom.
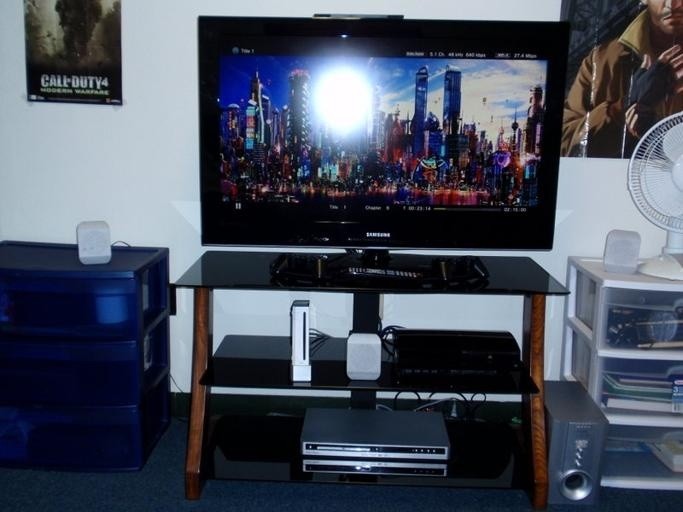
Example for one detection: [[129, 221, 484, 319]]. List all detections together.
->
[[171, 250, 572, 512]]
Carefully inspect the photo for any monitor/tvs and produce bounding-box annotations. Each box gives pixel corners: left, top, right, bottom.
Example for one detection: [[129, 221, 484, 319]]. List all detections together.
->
[[197, 15, 573, 290]]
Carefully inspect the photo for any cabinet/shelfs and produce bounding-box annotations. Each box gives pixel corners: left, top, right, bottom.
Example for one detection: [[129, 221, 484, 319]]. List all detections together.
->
[[560, 257, 683, 491], [1, 240, 171, 473]]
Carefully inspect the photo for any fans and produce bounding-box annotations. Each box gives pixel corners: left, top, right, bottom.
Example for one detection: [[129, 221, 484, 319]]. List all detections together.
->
[[627, 111, 683, 280]]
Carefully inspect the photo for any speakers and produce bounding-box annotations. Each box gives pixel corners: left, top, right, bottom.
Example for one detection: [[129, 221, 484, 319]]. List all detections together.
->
[[603, 228, 642, 277], [542, 379, 609, 507], [344, 331, 383, 381], [76, 220, 113, 266]]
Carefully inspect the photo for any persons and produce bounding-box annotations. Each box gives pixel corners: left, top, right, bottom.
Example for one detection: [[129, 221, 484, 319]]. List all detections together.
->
[[51, 0, 104, 72], [559, 1, 682, 159]]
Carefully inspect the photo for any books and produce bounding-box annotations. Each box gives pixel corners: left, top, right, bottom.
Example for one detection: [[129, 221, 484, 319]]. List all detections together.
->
[[597, 367, 683, 474]]
